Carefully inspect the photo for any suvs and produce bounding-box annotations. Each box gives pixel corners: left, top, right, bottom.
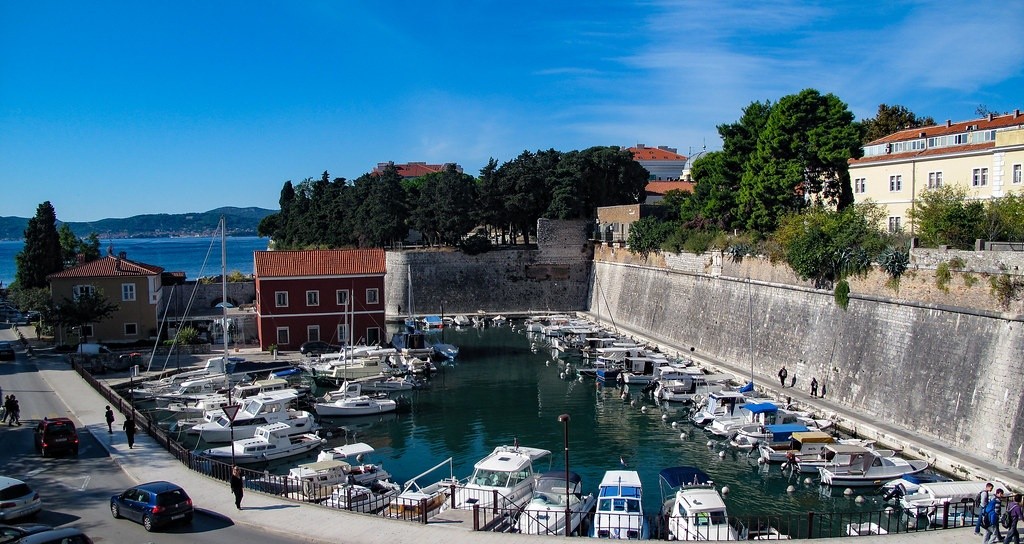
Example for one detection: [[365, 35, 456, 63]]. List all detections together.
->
[[300, 341, 342, 356], [24, 311, 41, 325], [32, 416, 79, 458], [110, 481, 195, 531]]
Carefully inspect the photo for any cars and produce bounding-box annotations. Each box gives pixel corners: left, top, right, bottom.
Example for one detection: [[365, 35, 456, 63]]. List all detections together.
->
[[0, 342, 16, 362], [0, 522, 90, 544], [0, 474, 42, 521]]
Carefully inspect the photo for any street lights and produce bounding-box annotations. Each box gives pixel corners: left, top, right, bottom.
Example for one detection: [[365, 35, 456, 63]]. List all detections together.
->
[[556, 413, 572, 536], [216, 388, 241, 505]]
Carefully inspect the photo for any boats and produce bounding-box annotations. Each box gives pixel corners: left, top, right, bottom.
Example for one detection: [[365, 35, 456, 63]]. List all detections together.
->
[[729, 417, 833, 448], [752, 525, 792, 540], [704, 403, 816, 438], [785, 439, 877, 472], [125, 213, 733, 443], [511, 469, 595, 536], [318, 473, 402, 513], [757, 432, 862, 461], [202, 421, 323, 464], [882, 473, 954, 498], [659, 465, 745, 541], [846, 521, 888, 536], [247, 441, 377, 503], [377, 455, 472, 523], [691, 391, 767, 424], [898, 479, 1024, 531], [592, 469, 645, 539], [816, 444, 937, 487], [438, 436, 553, 533]]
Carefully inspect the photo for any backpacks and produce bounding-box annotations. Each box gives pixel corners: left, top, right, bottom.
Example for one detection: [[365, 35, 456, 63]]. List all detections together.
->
[[980, 506, 995, 529], [974, 489, 988, 508], [1001, 505, 1021, 528]]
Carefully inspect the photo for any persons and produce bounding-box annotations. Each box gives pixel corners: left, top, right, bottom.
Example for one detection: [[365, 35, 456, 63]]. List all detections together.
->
[[982, 500, 998, 544], [0, 393, 23, 427], [123, 415, 134, 449], [973, 482, 994, 536], [810, 377, 818, 399], [230, 466, 243, 511], [1002, 493, 1024, 544], [105, 406, 114, 434], [35, 325, 42, 340], [982, 489, 1005, 540], [778, 366, 787, 388], [398, 305, 402, 316]]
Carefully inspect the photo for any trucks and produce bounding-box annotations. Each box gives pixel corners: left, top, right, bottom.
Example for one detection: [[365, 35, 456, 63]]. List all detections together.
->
[[77, 344, 112, 358]]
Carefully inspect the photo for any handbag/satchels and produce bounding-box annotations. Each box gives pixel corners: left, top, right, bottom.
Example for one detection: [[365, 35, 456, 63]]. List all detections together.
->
[[134, 426, 138, 433], [974, 507, 983, 515]]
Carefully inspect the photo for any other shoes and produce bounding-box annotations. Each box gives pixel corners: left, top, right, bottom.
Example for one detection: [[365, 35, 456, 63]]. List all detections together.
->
[[8, 422, 15, 427], [18, 422, 22, 426], [1000, 536, 1006, 541], [975, 531, 983, 537]]
